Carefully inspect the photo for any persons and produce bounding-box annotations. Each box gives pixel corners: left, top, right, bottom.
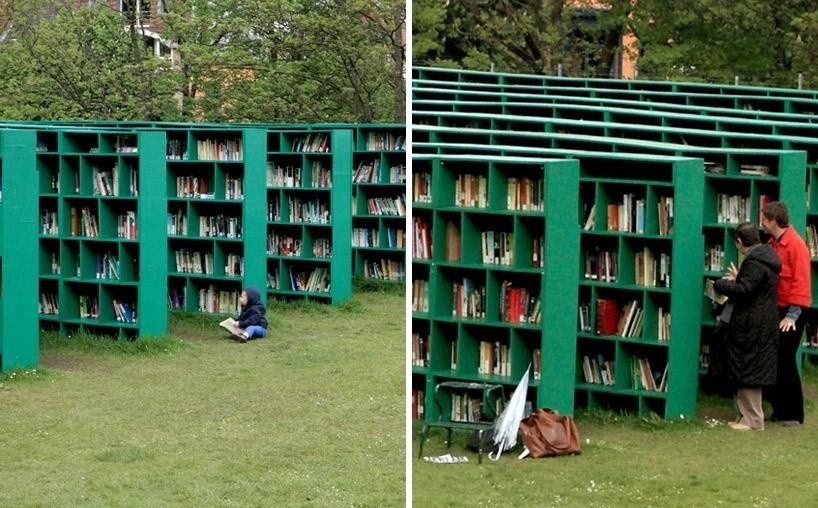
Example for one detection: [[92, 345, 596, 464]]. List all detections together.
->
[[230, 288, 269, 343], [713, 201, 811, 432]]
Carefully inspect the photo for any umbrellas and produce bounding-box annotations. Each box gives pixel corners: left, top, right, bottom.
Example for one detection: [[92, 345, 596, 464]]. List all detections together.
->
[[486, 364, 533, 462]]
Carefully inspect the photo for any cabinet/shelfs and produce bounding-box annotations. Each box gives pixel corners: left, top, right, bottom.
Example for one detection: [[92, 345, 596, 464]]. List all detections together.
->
[[411, 64, 817, 443], [0, 120, 405, 380]]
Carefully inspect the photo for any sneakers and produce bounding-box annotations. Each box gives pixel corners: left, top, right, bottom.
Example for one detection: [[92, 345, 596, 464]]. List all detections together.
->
[[233, 333, 248, 343], [728, 415, 800, 430]]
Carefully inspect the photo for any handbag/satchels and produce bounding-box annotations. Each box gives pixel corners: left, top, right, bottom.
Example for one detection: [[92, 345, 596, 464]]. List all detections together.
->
[[518, 407, 582, 458]]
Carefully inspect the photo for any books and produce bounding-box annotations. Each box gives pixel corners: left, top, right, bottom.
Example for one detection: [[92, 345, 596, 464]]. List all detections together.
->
[[219, 320, 243, 341]]
